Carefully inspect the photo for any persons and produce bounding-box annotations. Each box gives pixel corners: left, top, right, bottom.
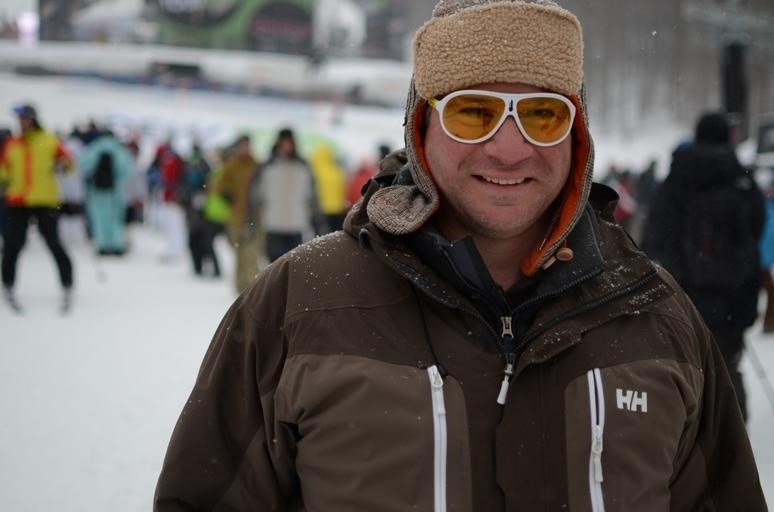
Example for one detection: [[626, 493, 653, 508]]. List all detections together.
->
[[151, 1, 768, 512], [607, 112, 774, 422], [0, 105, 389, 323]]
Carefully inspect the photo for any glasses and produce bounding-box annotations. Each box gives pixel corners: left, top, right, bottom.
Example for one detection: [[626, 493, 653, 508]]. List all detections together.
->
[[425, 90, 579, 148]]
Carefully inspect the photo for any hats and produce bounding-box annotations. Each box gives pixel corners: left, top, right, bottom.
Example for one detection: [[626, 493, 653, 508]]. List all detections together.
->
[[366, 0, 595, 280]]
